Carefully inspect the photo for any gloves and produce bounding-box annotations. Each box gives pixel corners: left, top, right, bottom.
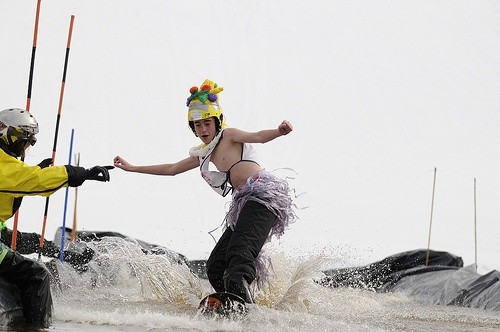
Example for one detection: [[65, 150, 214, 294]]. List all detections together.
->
[[38, 158, 53, 169], [64, 164, 115, 188]]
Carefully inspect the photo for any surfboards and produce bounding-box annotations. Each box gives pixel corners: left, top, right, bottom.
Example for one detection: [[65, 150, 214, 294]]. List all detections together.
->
[[198, 292, 248, 317]]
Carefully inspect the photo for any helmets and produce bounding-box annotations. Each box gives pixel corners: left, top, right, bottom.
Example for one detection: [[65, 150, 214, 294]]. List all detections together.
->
[[187, 97, 224, 137], [0, 108, 39, 162]]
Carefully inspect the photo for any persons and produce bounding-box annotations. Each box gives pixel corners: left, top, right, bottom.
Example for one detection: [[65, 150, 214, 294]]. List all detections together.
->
[[0, 109, 115, 331], [115, 93, 296, 323]]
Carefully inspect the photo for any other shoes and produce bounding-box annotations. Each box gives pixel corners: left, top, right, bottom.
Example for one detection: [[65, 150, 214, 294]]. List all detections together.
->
[[70, 247, 93, 274]]
